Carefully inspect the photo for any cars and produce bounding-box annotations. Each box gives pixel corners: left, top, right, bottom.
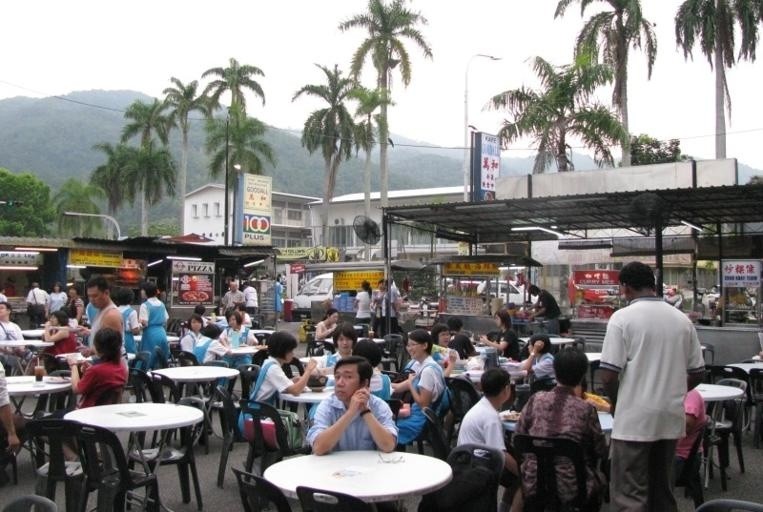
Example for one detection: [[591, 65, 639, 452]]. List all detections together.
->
[[702, 284, 753, 308], [477, 280, 541, 307], [449, 281, 481, 293]]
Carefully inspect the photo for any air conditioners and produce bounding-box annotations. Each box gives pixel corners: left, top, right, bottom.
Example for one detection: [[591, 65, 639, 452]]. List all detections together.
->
[[333, 217, 343, 225]]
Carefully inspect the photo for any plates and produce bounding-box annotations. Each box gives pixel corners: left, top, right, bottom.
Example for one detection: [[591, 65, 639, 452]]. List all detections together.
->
[[449, 369, 463, 374], [54, 326, 81, 332], [45, 376, 72, 384], [490, 298, 504, 317], [502, 416, 518, 421]]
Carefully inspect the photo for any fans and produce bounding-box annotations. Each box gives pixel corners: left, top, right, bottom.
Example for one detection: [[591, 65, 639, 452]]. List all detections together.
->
[[351, 213, 387, 245]]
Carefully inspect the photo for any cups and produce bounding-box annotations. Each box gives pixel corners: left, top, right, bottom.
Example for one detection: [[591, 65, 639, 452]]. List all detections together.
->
[[209, 313, 216, 324], [368, 331, 374, 340], [231, 331, 239, 348], [34, 365, 44, 381]]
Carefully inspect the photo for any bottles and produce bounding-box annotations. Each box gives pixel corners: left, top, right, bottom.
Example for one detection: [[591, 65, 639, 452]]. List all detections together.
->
[[484, 348, 497, 369]]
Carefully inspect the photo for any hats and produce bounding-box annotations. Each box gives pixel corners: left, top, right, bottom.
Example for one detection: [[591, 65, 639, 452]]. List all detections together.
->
[[31, 282, 41, 287]]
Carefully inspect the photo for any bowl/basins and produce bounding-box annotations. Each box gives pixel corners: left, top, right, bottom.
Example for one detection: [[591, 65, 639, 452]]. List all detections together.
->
[[697, 318, 710, 325], [306, 374, 328, 391]]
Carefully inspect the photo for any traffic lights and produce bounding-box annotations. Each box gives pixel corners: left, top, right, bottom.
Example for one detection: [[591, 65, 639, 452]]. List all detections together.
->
[[7, 200, 24, 208]]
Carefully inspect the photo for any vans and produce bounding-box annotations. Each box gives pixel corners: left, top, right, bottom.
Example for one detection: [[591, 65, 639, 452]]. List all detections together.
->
[[293, 271, 402, 309]]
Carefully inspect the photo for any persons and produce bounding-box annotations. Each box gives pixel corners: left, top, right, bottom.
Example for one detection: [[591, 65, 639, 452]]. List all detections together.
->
[[0, 273, 745, 474], [598, 262, 706, 510], [509, 346, 606, 511], [673, 389, 706, 486], [456, 367, 525, 509]]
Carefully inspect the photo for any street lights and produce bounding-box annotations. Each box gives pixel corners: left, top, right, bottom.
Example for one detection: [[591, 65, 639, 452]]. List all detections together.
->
[[63, 211, 121, 240], [462, 48, 505, 204], [232, 161, 244, 246]]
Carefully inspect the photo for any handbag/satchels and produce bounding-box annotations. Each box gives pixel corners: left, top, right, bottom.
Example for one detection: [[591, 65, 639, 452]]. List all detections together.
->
[[273, 408, 304, 450]]
